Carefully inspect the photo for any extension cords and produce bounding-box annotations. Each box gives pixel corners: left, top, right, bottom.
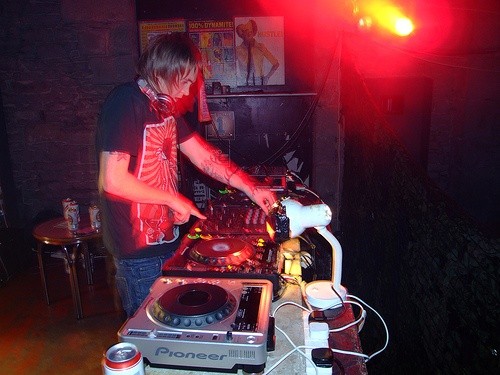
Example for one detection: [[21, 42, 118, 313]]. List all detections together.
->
[[303, 310, 333, 375]]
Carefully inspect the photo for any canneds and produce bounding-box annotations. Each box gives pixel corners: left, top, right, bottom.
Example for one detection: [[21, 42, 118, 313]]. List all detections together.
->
[[102, 341, 146, 375], [62, 198, 103, 233]]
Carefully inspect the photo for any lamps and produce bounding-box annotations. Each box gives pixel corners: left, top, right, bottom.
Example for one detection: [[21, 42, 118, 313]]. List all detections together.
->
[[278, 198, 347, 310]]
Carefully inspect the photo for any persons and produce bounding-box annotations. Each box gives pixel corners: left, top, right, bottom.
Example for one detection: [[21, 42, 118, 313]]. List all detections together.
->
[[94, 33, 279, 315], [233, 20, 279, 86]]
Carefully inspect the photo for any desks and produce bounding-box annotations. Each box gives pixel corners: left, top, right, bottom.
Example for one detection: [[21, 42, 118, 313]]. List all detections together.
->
[[32, 216, 117, 320]]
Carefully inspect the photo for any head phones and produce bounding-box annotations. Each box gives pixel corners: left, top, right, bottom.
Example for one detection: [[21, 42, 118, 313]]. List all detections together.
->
[[137, 77, 175, 118]]
